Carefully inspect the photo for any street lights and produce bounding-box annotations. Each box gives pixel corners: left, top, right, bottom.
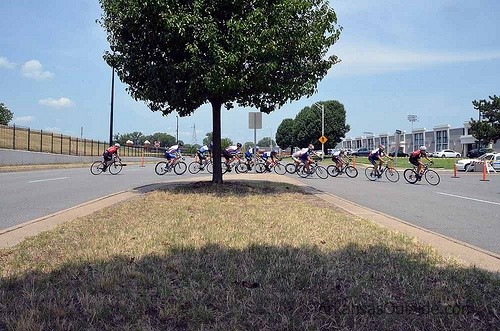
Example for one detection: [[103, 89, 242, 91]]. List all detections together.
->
[[396, 130, 402, 150], [176, 115, 179, 145], [108, 44, 118, 158], [406, 113, 420, 153]]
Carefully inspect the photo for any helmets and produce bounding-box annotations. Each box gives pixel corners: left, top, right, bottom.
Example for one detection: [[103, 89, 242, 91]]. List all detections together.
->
[[340, 149, 345, 152], [273, 149, 278, 153], [237, 142, 242, 146], [210, 141, 213, 146], [379, 145, 385, 148], [115, 143, 120, 148], [309, 144, 314, 149], [420, 146, 426, 150], [178, 142, 183, 146]]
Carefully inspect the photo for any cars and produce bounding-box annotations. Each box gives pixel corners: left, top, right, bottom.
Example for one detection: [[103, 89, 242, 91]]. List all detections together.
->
[[317, 148, 351, 155], [351, 147, 372, 157], [456, 151, 500, 172], [432, 149, 461, 158], [226, 146, 243, 158]]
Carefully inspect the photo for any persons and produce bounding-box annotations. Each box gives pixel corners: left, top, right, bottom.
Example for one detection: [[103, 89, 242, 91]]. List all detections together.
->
[[102, 143, 120, 172], [165, 142, 351, 175], [368, 144, 395, 177], [408, 146, 435, 179]]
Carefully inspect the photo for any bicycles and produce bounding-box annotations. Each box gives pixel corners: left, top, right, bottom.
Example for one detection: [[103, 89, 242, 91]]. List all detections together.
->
[[403, 161, 440, 186], [90, 155, 122, 176], [155, 157, 249, 176], [327, 157, 359, 178], [364, 159, 400, 182], [255, 159, 287, 175], [285, 158, 328, 180]]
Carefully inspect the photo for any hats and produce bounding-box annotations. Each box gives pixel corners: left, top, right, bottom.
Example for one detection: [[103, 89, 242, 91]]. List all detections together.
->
[[301, 148, 309, 154]]
